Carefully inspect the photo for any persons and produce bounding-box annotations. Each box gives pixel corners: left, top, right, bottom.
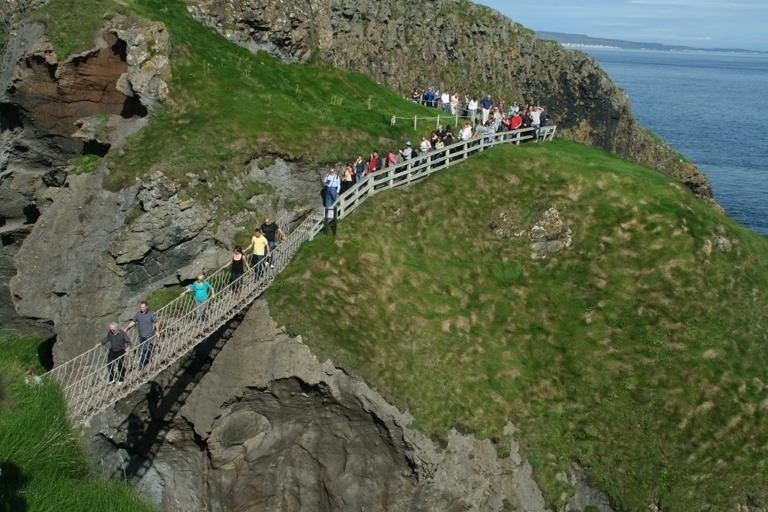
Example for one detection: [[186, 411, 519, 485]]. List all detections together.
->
[[179, 274, 216, 326], [22, 366, 44, 386], [121, 300, 162, 373], [240, 228, 270, 283], [94, 321, 133, 386], [318, 84, 551, 211], [227, 244, 251, 300], [259, 218, 286, 270]]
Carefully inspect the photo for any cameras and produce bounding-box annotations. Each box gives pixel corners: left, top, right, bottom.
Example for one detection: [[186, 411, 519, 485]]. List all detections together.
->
[[326, 180, 331, 185]]
